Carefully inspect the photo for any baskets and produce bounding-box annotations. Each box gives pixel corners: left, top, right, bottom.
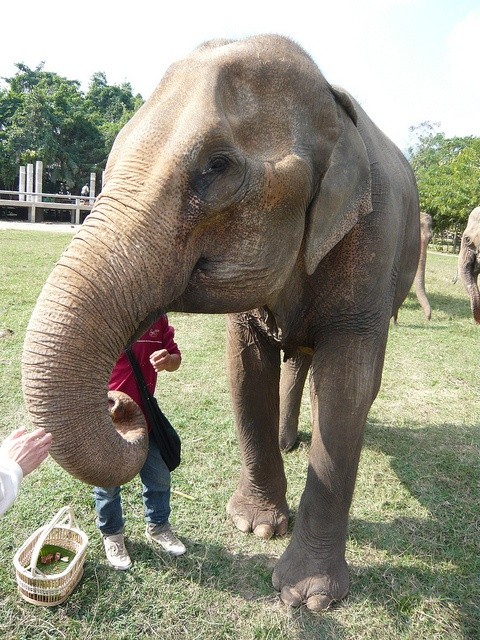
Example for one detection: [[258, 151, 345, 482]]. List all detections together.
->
[[13, 506, 88, 607]]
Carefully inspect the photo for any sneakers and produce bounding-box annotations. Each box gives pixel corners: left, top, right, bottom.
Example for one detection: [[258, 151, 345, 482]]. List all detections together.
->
[[103, 534, 132, 570], [145, 523, 186, 556]]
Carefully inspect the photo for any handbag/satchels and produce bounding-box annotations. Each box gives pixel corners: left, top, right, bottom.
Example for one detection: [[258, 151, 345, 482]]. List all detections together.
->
[[126, 348, 181, 472]]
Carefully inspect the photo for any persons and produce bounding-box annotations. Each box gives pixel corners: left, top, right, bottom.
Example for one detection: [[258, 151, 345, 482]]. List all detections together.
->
[[92, 313, 187, 572], [0, 425, 53, 519]]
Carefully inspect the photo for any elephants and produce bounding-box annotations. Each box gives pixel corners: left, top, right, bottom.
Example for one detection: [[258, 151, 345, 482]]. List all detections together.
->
[[452, 207, 480, 325], [20, 35, 421, 612], [392, 211, 433, 326]]
